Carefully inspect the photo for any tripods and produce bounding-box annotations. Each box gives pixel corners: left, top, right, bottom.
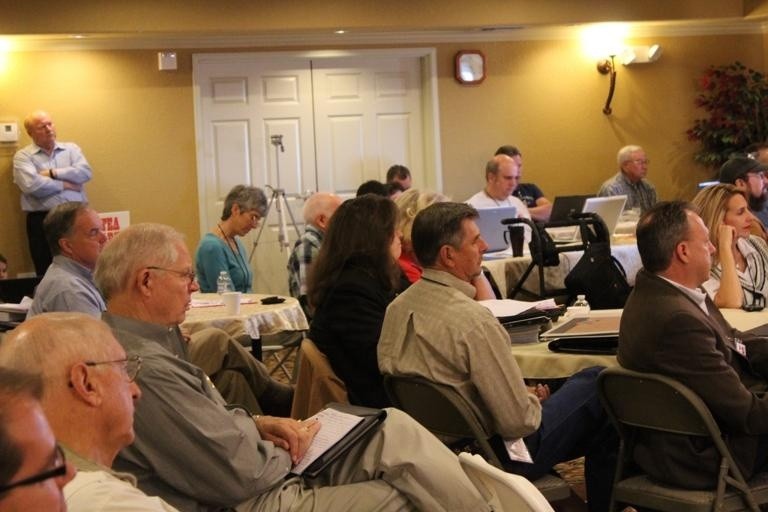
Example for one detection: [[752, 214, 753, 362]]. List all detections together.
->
[[248, 148, 303, 267]]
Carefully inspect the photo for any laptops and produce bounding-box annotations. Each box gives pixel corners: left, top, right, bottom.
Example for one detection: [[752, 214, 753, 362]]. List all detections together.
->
[[468, 207, 517, 254], [553, 195, 627, 244], [548, 194, 596, 225]]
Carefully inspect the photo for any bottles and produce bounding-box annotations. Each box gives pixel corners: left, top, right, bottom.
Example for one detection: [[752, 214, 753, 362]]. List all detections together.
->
[[216, 269, 236, 297], [572, 296, 590, 312]]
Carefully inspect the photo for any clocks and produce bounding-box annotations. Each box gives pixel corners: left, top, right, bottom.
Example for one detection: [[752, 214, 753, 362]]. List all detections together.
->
[[455, 50, 487, 86]]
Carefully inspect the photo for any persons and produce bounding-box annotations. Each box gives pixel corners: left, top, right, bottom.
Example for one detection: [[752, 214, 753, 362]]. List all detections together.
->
[[460, 156, 533, 258], [308, 195, 411, 409], [377, 201, 627, 512], [354, 163, 410, 201], [496, 145, 555, 225], [0, 367, 77, 512], [0, 311, 183, 512], [717, 157, 768, 235], [91, 221, 490, 511], [617, 200, 768, 490], [23, 200, 107, 321], [286, 192, 344, 319], [597, 145, 662, 215], [194, 183, 267, 292], [392, 189, 496, 301], [688, 184, 768, 312], [11, 111, 92, 278]]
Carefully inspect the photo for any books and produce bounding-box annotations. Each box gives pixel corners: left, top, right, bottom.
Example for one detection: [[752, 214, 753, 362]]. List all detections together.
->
[[1, 296, 33, 322], [290, 407, 365, 476], [475, 298, 565, 343], [539, 315, 622, 338]]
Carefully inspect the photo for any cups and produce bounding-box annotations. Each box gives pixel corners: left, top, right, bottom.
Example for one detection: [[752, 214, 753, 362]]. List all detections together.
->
[[222, 291, 241, 317]]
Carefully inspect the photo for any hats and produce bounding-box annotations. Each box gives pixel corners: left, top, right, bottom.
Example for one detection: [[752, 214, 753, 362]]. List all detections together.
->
[[719, 157, 768, 182]]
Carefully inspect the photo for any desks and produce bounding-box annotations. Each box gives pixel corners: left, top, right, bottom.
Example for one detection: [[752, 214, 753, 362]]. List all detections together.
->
[[482, 238, 639, 299], [516, 304, 768, 380], [181, 291, 309, 362]]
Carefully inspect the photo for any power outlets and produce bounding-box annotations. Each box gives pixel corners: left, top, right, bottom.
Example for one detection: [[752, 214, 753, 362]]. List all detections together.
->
[[157, 51, 178, 71]]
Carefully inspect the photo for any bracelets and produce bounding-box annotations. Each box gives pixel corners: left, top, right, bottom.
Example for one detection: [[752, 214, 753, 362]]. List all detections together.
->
[[49, 168, 55, 180]]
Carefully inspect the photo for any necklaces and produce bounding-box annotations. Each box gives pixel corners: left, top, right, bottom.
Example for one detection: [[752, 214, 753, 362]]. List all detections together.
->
[[734, 251, 743, 270]]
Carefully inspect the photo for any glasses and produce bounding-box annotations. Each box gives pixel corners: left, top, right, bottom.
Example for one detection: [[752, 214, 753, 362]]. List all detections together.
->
[[68, 352, 143, 390], [745, 173, 766, 180], [0, 446, 68, 491], [630, 158, 651, 166], [243, 215, 262, 225], [146, 264, 197, 284]]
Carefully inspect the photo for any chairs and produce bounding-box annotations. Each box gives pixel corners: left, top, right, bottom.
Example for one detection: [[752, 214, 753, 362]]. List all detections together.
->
[[385, 373, 570, 504], [292, 337, 345, 413], [595, 367, 768, 511]]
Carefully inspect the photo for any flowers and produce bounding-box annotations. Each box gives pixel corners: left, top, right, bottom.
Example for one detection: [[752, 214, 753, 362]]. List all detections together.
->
[[685, 60, 768, 159]]
